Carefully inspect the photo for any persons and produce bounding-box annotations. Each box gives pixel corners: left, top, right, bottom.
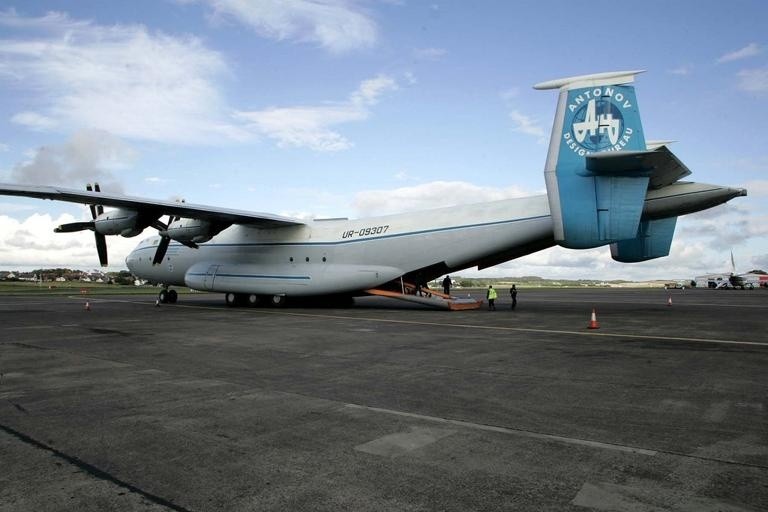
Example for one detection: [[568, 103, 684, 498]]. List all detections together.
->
[[486, 285, 498, 312], [441, 275, 453, 298], [509, 284, 517, 311]]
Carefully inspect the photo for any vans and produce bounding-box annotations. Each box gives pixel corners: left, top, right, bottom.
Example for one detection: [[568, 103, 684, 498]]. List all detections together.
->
[[664, 281, 683, 289]]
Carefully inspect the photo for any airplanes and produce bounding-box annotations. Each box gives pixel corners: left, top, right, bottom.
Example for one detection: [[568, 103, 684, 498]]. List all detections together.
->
[[0, 68, 749, 315], [703, 251, 767, 291]]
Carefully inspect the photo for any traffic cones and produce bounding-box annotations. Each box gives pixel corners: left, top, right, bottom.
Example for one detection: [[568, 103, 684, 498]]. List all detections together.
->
[[586, 308, 601, 330], [84, 299, 91, 312], [667, 292, 672, 306]]
